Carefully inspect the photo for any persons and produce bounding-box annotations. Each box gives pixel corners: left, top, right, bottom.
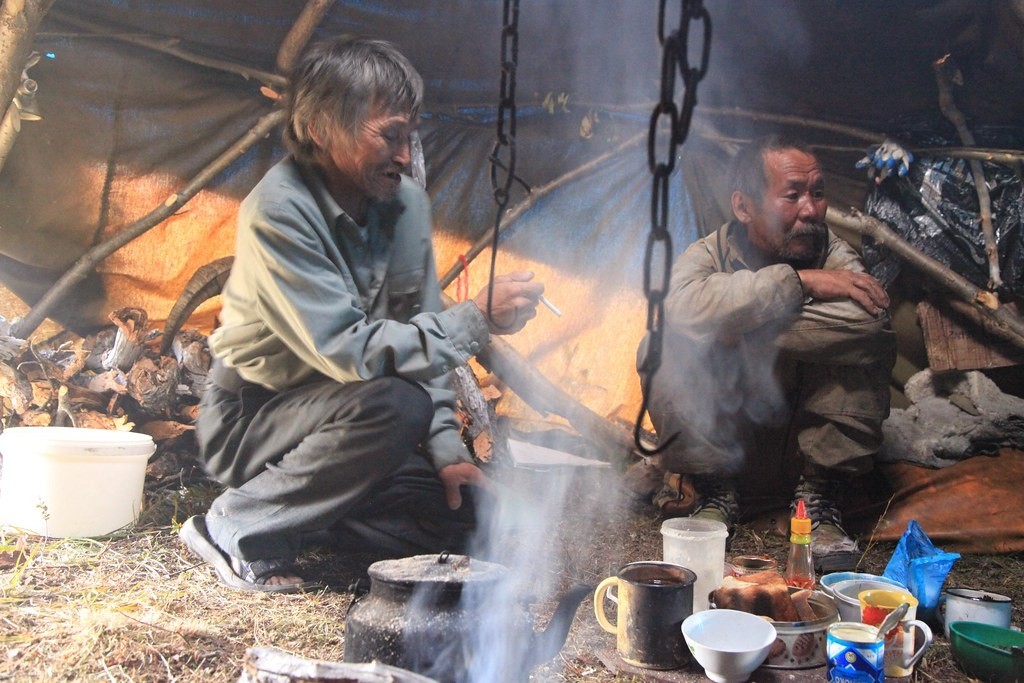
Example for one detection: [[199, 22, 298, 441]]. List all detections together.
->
[[635, 133, 898, 573], [178, 32, 543, 594]]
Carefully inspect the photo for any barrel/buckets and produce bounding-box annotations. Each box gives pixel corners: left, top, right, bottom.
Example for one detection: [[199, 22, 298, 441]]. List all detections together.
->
[[0, 426, 157, 538]]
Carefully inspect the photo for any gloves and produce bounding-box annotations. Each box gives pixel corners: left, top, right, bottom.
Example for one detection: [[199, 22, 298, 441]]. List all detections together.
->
[[855, 138, 913, 183]]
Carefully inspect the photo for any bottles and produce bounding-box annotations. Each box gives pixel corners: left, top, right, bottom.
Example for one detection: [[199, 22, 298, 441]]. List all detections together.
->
[[784, 500, 816, 590]]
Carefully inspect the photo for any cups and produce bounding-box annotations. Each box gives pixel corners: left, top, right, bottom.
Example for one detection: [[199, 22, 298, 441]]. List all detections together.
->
[[858, 590, 933, 678], [660, 517, 729, 613], [594, 561, 696, 670], [934, 587, 1012, 642]]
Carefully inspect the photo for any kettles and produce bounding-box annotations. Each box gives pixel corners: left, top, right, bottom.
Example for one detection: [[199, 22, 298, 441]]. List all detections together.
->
[[342, 551, 593, 682]]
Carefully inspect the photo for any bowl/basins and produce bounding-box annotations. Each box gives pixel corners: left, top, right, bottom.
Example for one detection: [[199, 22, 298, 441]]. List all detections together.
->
[[681, 609, 778, 683], [832, 579, 913, 624], [949, 622, 1024, 683], [819, 572, 908, 596]]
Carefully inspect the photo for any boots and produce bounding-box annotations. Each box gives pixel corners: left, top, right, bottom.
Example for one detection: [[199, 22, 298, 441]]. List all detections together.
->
[[686, 486, 739, 540], [786, 463, 864, 571]]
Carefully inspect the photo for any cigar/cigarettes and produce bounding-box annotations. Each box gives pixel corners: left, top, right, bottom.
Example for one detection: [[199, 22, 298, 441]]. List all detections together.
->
[[539, 293, 562, 318]]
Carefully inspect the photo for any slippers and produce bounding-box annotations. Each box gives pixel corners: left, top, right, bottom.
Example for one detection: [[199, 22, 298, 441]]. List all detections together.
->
[[179, 515, 321, 593]]
